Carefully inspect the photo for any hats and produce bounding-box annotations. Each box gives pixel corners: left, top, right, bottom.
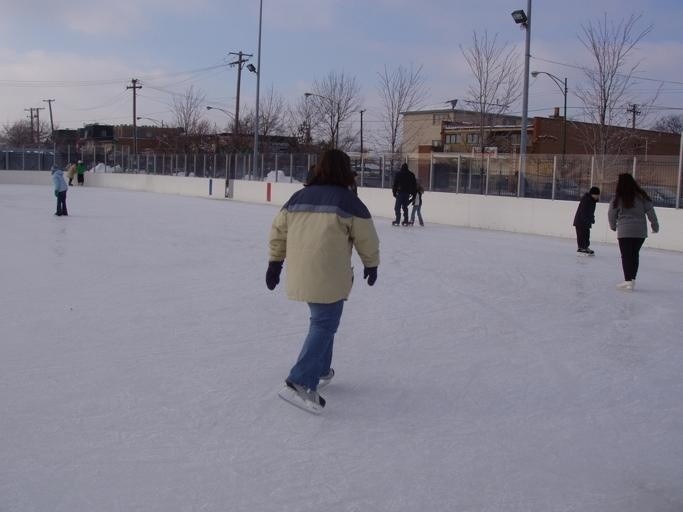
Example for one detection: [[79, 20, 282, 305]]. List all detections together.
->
[[590, 187, 600, 195], [51, 165, 58, 171], [401, 163, 408, 169]]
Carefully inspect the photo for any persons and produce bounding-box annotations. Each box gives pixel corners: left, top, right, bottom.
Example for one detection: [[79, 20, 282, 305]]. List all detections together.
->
[[265, 150, 380, 408], [67, 159, 77, 186], [393, 163, 424, 225], [51, 166, 67, 215], [573, 186, 600, 254], [77, 160, 85, 186], [608, 173, 660, 290]]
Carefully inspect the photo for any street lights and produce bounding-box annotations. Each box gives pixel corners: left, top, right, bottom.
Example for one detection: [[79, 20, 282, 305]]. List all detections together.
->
[[531, 71, 568, 177], [304, 93, 339, 149], [511, 9, 531, 197], [247, 64, 260, 176], [137, 116, 168, 128], [206, 105, 235, 123]]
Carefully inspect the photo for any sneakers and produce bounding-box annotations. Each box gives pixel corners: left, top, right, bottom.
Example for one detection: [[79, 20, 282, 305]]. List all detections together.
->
[[576, 248, 594, 254], [408, 222, 424, 227], [286, 380, 327, 408], [616, 279, 636, 290], [402, 221, 408, 224], [392, 221, 400, 224], [68, 184, 73, 186], [319, 368, 335, 379], [55, 211, 67, 215]]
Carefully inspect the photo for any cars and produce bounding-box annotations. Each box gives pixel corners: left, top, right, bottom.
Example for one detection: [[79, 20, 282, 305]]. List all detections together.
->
[[541, 178, 588, 201], [602, 185, 683, 208]]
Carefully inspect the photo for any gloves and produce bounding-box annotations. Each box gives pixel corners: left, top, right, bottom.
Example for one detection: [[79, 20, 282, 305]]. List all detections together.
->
[[265, 260, 283, 291], [363, 266, 378, 287]]
